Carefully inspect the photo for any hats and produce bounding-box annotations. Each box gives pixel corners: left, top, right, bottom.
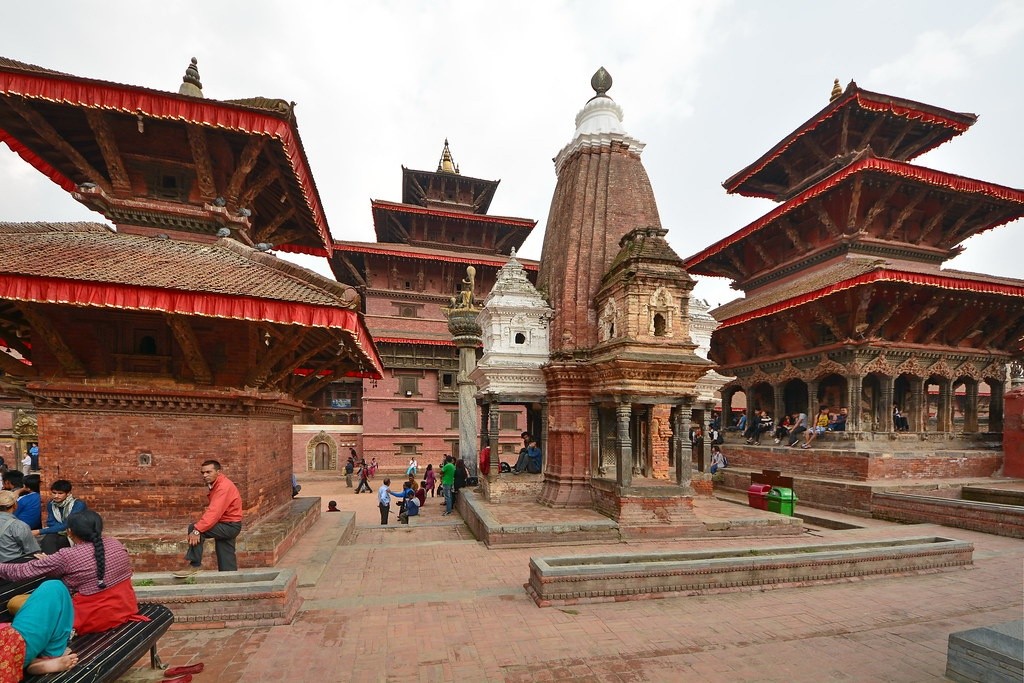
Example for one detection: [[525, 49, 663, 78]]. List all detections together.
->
[[0, 489, 19, 507]]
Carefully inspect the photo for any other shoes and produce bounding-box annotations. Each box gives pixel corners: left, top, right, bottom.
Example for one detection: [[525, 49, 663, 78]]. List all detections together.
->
[[725, 425, 834, 449], [441, 511, 451, 516], [397, 515, 401, 521], [345, 475, 377, 494], [512, 464, 517, 468], [158, 673, 192, 683], [899, 426, 909, 431], [441, 502, 447, 505], [164, 663, 204, 677], [513, 469, 524, 474]]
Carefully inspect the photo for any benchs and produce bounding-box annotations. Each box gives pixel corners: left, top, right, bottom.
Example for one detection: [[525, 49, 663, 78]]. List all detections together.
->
[[1, 551, 176, 683]]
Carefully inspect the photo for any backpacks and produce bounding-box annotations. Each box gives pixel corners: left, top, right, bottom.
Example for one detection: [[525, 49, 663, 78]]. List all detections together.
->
[[717, 432, 724, 444], [718, 452, 727, 468]]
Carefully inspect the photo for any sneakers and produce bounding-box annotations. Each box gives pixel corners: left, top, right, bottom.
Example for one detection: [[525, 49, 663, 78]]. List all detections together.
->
[[173, 564, 203, 578]]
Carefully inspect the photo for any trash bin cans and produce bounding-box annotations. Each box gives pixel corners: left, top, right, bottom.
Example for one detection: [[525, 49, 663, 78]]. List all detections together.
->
[[766, 485, 799, 517], [747, 483, 772, 511]]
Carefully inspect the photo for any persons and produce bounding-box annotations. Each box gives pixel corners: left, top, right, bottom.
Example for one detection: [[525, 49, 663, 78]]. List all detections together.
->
[[774, 413, 791, 444], [387, 453, 472, 525], [0, 578, 79, 683], [170, 460, 244, 578], [707, 445, 728, 474], [29, 442, 40, 471], [31, 480, 92, 557], [827, 406, 849, 432], [375, 477, 392, 525], [20, 452, 32, 477], [0, 455, 30, 501], [324, 501, 341, 512], [786, 411, 808, 446], [501, 428, 542, 477], [292, 472, 301, 497], [801, 405, 837, 449], [0, 489, 41, 564], [685, 403, 774, 448], [341, 446, 380, 493], [12, 473, 41, 530], [478, 438, 501, 480], [892, 400, 910, 432], [0, 508, 136, 630]]
